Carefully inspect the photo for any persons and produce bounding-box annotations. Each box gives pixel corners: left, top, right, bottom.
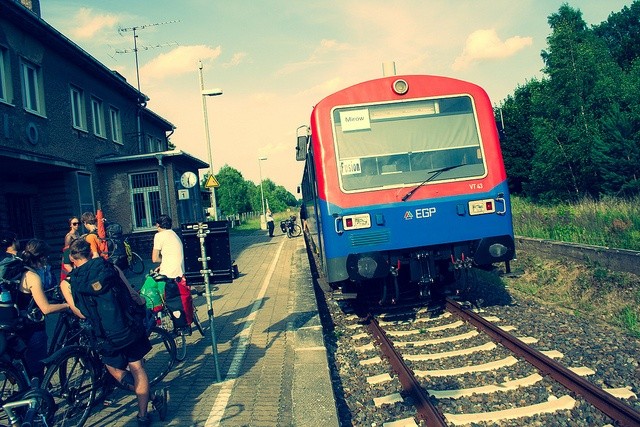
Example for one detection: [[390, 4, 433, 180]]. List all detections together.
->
[[61, 216, 82, 248], [81, 211, 110, 259], [151, 214, 185, 278], [0, 237, 69, 385], [59, 240, 167, 427], [60, 248, 74, 282], [300, 204, 307, 231], [266, 208, 275, 236], [2, 229, 20, 258]]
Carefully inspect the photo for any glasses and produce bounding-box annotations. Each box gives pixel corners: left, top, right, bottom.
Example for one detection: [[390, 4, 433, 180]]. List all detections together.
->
[[71, 222, 80, 226]]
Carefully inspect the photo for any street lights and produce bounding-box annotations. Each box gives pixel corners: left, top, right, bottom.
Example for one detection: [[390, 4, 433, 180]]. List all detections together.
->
[[259, 156, 268, 230], [196, 59, 225, 220]]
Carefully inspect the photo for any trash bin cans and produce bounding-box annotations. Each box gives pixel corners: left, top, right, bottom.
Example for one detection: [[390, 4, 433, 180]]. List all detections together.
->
[[181, 221, 238, 284]]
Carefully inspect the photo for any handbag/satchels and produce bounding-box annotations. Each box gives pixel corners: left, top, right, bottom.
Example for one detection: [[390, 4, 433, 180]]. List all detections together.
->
[[139, 273, 168, 310]]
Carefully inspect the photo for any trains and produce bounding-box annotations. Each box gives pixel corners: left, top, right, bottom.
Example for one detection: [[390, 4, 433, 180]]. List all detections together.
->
[[297, 75, 514, 320]]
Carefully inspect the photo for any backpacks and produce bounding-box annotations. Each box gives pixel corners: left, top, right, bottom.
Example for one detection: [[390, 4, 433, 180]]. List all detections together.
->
[[34, 255, 58, 300], [155, 274, 194, 328], [0, 253, 40, 357], [87, 220, 132, 271], [61, 256, 146, 357]]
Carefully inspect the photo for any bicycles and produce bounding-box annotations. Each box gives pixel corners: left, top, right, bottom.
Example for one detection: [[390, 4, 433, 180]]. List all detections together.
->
[[36, 318, 177, 425], [108, 235, 145, 276], [146, 269, 205, 363], [1, 353, 55, 427]]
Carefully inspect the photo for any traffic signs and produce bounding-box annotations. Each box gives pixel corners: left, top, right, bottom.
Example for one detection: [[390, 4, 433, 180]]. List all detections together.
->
[[204, 173, 221, 188]]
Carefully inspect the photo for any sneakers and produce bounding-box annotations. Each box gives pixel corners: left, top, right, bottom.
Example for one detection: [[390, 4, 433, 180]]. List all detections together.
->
[[137, 413, 153, 427], [103, 395, 115, 406], [151, 388, 167, 421], [60, 388, 71, 399]]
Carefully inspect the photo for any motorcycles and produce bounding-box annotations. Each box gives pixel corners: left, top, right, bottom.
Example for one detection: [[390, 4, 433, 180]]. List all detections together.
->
[[279, 214, 301, 239]]
